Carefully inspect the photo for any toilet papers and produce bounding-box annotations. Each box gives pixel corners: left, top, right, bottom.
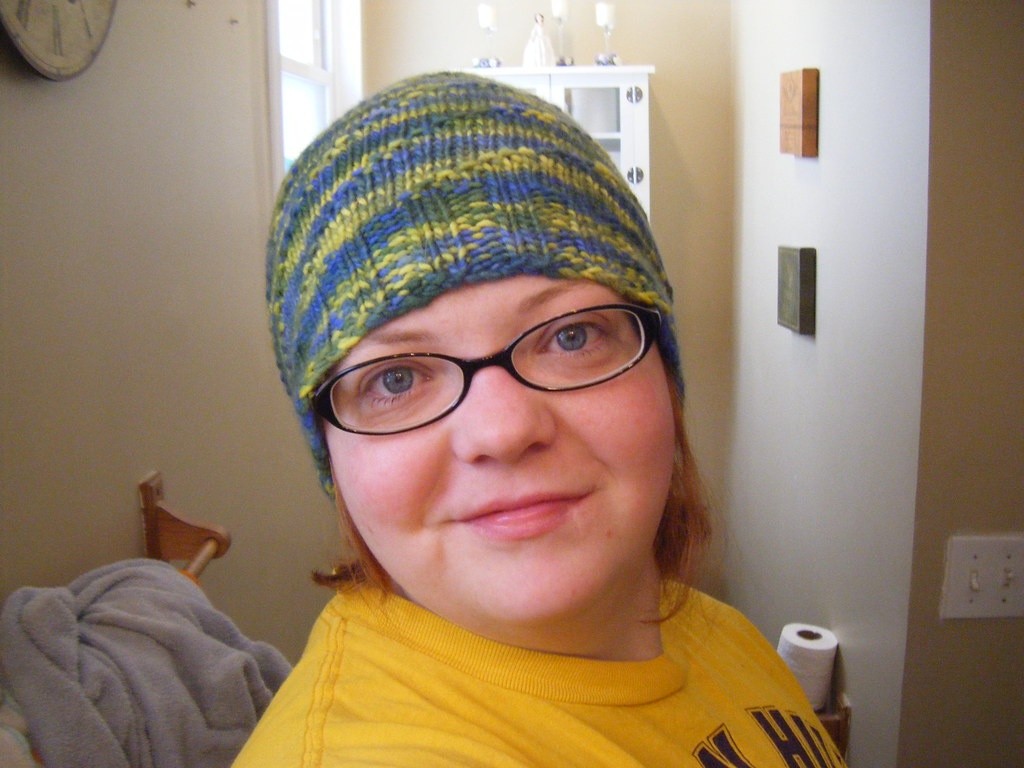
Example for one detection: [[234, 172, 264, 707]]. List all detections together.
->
[[771, 622, 841, 713]]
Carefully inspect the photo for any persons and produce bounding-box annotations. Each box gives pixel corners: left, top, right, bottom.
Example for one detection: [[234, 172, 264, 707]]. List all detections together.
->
[[229, 71, 851, 768]]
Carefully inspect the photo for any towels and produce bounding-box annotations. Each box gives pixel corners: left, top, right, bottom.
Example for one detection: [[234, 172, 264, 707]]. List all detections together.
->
[[2, 555, 292, 768]]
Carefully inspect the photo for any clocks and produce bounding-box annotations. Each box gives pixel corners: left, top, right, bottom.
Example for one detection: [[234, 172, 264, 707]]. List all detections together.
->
[[0, 0, 119, 82]]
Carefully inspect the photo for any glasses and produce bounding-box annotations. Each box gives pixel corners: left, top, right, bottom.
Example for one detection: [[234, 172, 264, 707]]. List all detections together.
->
[[308, 302, 666, 436]]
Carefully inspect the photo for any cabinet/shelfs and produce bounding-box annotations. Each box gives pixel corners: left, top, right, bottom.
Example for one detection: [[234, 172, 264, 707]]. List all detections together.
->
[[465, 63, 656, 229]]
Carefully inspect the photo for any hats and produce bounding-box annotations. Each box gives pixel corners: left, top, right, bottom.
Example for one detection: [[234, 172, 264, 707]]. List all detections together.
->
[[264, 71, 687, 513]]
